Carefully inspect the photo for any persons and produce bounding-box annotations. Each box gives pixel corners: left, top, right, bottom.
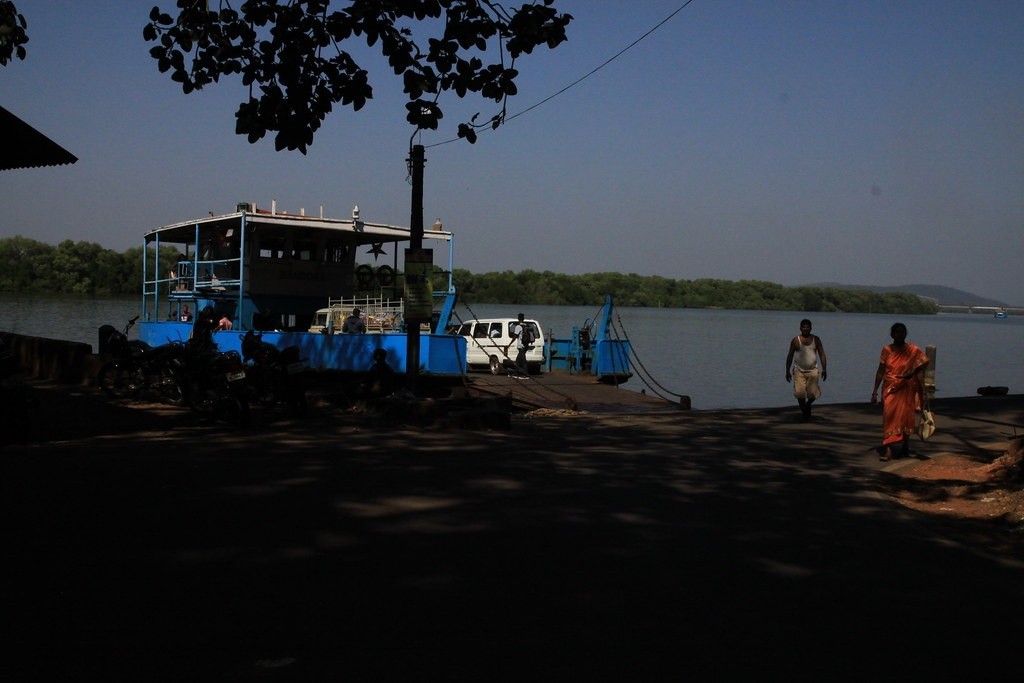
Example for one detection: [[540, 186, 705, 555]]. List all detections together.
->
[[872, 323, 930, 462], [786, 319, 827, 419], [447, 324, 501, 338], [192, 306, 220, 400], [342, 308, 366, 334], [506, 313, 530, 380], [356, 349, 394, 402], [168, 255, 233, 331]]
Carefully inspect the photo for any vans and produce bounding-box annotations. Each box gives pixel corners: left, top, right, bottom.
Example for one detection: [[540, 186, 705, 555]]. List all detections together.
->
[[453, 318, 547, 376]]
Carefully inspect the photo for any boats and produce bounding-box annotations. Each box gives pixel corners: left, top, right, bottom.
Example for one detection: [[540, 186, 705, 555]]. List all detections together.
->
[[993, 312, 1008, 319]]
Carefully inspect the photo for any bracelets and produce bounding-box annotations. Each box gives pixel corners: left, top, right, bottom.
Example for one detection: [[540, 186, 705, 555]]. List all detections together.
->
[[872, 393, 878, 396], [822, 370, 826, 372]]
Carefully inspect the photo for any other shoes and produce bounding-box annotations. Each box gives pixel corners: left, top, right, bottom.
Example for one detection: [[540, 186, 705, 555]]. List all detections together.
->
[[512, 374, 530, 379]]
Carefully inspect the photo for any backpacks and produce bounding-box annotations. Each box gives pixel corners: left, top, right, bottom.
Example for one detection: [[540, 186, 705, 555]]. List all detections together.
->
[[520, 324, 535, 344]]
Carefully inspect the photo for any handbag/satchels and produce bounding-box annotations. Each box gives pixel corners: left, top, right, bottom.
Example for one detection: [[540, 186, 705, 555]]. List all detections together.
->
[[918, 401, 935, 441]]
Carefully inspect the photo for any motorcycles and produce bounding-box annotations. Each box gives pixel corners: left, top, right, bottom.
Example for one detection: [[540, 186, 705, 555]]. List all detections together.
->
[[97, 315, 313, 428]]
